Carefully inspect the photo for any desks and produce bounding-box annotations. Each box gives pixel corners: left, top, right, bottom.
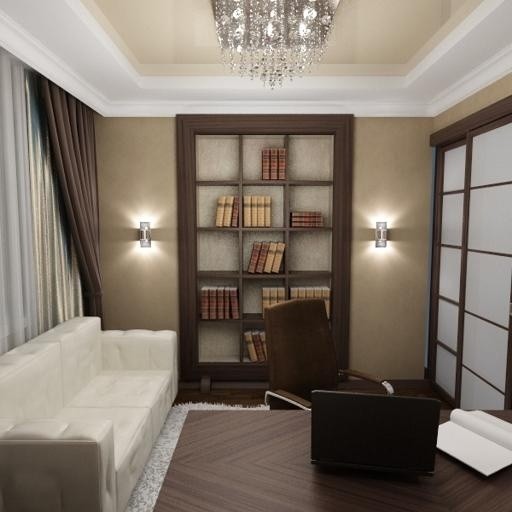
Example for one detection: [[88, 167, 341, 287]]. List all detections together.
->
[[153, 409, 511, 511]]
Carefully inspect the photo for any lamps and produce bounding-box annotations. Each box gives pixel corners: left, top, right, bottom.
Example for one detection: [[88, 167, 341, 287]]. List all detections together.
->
[[375, 221, 387, 248], [210, 0, 341, 92], [139, 221, 152, 248]]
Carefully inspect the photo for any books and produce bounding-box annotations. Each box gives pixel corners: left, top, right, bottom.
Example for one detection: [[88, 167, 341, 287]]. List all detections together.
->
[[261, 147, 270, 180], [270, 147, 278, 179], [434, 407, 512, 477], [198, 194, 332, 363], [278, 147, 286, 180]]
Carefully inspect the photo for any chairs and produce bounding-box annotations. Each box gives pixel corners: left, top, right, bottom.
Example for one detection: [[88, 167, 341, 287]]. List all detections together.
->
[[262, 298, 396, 410]]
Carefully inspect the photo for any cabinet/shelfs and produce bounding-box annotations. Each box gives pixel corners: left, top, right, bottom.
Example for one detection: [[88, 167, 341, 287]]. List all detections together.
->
[[175, 113, 355, 393]]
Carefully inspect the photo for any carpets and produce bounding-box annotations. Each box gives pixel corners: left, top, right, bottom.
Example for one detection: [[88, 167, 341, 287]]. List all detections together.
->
[[123, 400, 272, 511]]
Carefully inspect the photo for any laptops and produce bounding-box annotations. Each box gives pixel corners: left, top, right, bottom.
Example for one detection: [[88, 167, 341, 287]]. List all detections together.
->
[[309, 390, 442, 477]]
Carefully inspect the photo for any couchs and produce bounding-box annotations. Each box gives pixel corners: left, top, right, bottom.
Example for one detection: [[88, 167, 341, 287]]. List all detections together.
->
[[0, 315, 178, 512]]
